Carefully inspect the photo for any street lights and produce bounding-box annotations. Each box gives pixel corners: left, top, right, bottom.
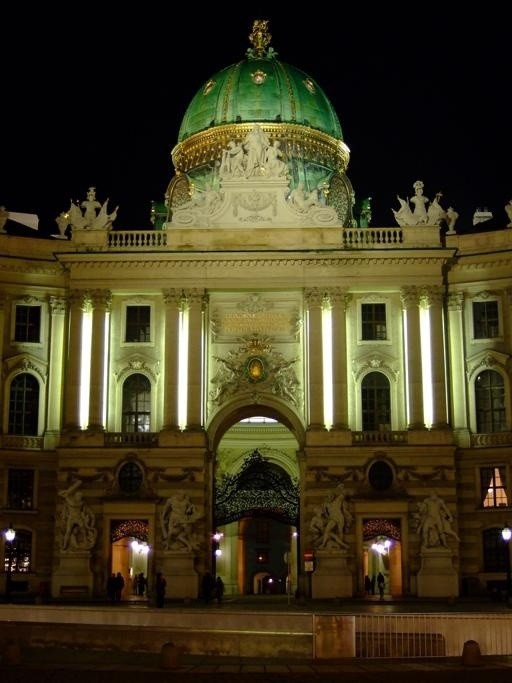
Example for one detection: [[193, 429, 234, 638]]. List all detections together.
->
[[6, 522, 15, 596], [502, 521, 511, 586]]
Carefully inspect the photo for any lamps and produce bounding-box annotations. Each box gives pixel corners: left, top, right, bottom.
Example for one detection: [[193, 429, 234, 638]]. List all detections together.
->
[[502, 521, 512, 543]]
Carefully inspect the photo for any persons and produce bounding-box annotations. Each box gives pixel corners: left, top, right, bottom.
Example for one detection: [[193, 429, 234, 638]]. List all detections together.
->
[[159, 489, 196, 553], [419, 490, 455, 550], [415, 502, 463, 546], [80, 186, 102, 220], [165, 489, 197, 553], [170, 182, 222, 212], [371, 576, 375, 595], [270, 358, 301, 407], [318, 489, 346, 551], [201, 572, 213, 606], [139, 573, 145, 595], [214, 577, 224, 604], [115, 572, 124, 600], [365, 575, 370, 596], [410, 181, 430, 225], [377, 572, 384, 595], [210, 355, 243, 407], [310, 505, 353, 552], [289, 188, 318, 212], [132, 575, 138, 594], [58, 488, 94, 550], [155, 572, 167, 604], [215, 124, 291, 179], [106, 573, 115, 601]]
